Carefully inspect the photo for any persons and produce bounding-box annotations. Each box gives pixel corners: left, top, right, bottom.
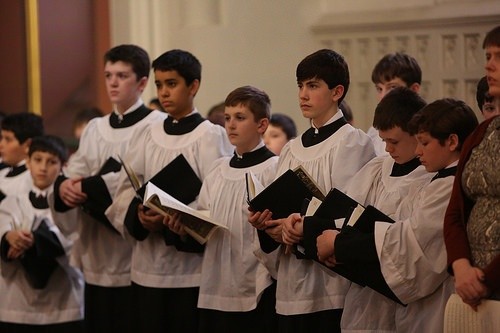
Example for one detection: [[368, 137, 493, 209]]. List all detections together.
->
[[476, 75, 500, 120], [104, 49, 236, 333], [316, 99, 479, 333], [367, 52, 422, 157], [163, 83, 280, 333], [241, 48, 378, 333], [149, 98, 167, 112], [263, 113, 297, 157], [0, 132, 86, 333], [311, 100, 353, 126], [73, 107, 102, 140], [0, 111, 46, 203], [47, 43, 163, 332], [208, 103, 227, 126], [282, 87, 438, 333], [443, 26, 500, 333]]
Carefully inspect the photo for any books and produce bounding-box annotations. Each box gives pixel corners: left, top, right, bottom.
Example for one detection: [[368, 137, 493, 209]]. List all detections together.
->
[[118, 154, 202, 208], [289, 186, 367, 288], [336, 203, 409, 309], [244, 164, 328, 231], [61, 156, 122, 232], [8, 213, 58, 290], [141, 181, 230, 244]]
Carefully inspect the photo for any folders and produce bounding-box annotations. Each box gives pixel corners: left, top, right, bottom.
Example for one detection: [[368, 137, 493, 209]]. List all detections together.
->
[[117, 153, 202, 206], [245, 169, 409, 307]]
